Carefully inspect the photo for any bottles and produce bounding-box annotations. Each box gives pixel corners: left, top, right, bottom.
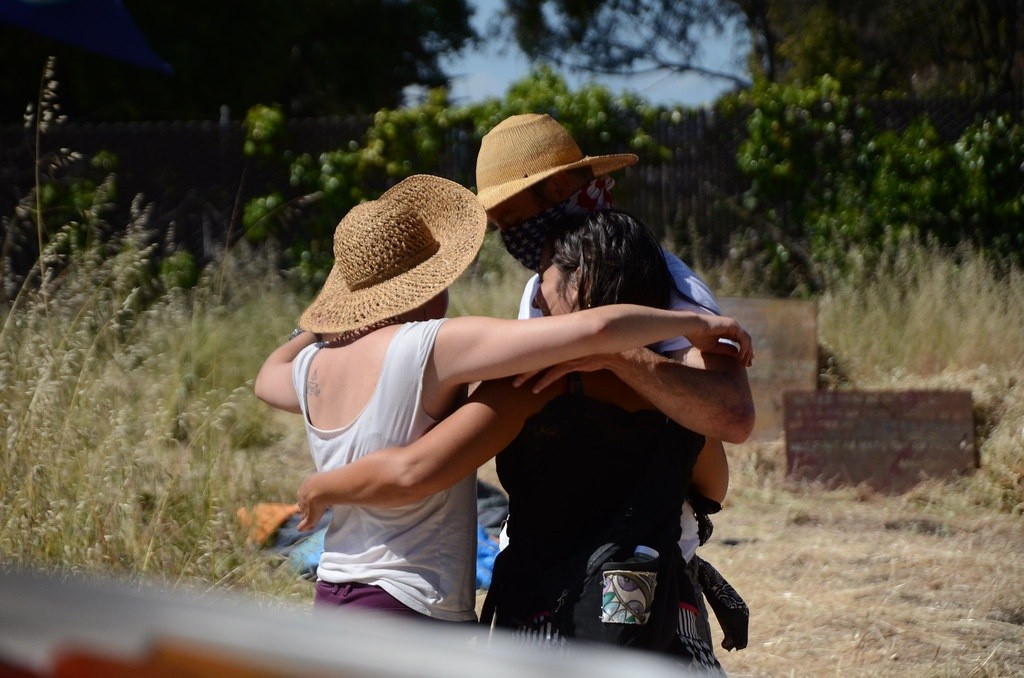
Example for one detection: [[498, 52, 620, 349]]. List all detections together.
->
[[626, 546, 659, 563]]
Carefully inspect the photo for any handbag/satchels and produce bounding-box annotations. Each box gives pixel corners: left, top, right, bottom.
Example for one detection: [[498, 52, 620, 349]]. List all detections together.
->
[[573, 542, 680, 650]]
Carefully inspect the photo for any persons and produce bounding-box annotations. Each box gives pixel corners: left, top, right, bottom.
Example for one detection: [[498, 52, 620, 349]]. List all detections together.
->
[[298, 209, 728, 676], [254, 174, 753, 625], [476, 113, 756, 650]]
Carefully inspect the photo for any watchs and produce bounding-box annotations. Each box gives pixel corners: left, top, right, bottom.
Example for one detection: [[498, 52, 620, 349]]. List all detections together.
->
[[288, 327, 304, 341]]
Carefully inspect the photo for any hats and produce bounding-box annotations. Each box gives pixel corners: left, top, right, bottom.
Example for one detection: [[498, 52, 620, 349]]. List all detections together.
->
[[298, 174, 487, 334], [476, 113, 639, 211]]
